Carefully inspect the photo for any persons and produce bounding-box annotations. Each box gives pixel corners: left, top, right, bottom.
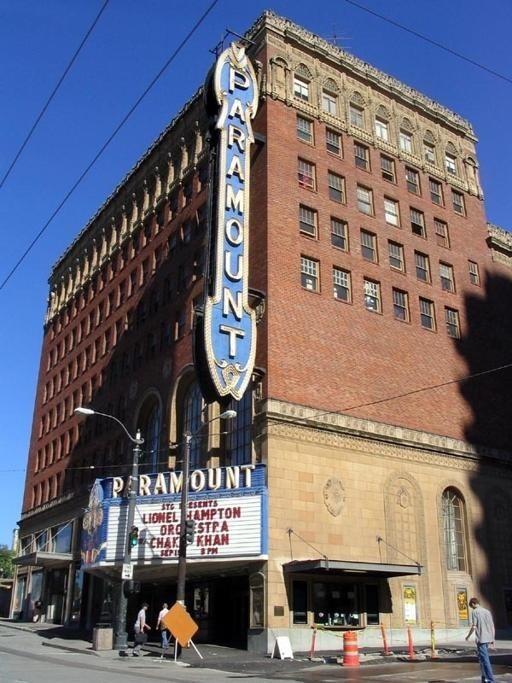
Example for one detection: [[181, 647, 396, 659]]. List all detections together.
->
[[133, 602, 152, 656], [464, 595, 499, 683], [155, 602, 170, 648]]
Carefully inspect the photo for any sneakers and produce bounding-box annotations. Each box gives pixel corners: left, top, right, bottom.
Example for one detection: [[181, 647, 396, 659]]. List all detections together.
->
[[132, 645, 170, 657]]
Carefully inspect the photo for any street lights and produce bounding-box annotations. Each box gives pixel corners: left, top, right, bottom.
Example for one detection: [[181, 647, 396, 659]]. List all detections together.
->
[[173, 410, 237, 658], [74, 407, 145, 649]]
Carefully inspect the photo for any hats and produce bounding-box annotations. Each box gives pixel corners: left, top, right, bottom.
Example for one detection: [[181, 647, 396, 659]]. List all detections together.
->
[[142, 602, 150, 607]]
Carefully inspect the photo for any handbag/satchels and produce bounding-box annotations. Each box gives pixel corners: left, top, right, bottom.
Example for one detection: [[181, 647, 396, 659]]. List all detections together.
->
[[135, 633, 147, 644]]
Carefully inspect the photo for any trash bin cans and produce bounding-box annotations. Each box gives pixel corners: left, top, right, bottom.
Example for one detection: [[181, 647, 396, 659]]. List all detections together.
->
[[92, 623, 113, 650]]
